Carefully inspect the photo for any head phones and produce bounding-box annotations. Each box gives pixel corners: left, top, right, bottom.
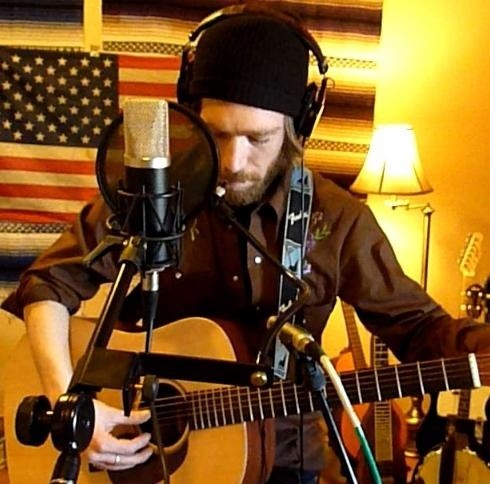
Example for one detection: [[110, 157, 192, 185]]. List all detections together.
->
[[177, 4, 328, 138]]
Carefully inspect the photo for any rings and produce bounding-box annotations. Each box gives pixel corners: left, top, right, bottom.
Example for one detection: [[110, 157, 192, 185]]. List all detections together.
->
[[113, 454, 121, 465]]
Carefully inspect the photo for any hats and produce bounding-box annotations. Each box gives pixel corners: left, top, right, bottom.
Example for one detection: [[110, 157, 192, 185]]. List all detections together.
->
[[191, 10, 310, 117]]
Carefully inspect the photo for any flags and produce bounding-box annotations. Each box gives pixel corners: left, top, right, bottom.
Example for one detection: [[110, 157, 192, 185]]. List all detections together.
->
[[1, 46, 194, 232]]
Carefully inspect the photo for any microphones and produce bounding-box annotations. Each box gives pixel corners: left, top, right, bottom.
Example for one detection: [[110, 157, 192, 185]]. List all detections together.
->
[[267, 315, 326, 367], [124, 99, 171, 320]]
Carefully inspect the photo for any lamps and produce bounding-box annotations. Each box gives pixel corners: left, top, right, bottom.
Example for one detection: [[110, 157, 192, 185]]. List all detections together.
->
[[349, 121, 435, 293]]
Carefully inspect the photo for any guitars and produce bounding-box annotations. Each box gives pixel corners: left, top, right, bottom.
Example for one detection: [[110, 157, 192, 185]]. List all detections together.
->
[[354, 334, 408, 484], [401, 233, 490, 484], [0, 314, 490, 484], [335, 298, 409, 458]]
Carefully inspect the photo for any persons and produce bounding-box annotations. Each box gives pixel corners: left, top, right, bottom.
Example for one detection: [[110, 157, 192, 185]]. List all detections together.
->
[[1, 3, 489, 483]]
